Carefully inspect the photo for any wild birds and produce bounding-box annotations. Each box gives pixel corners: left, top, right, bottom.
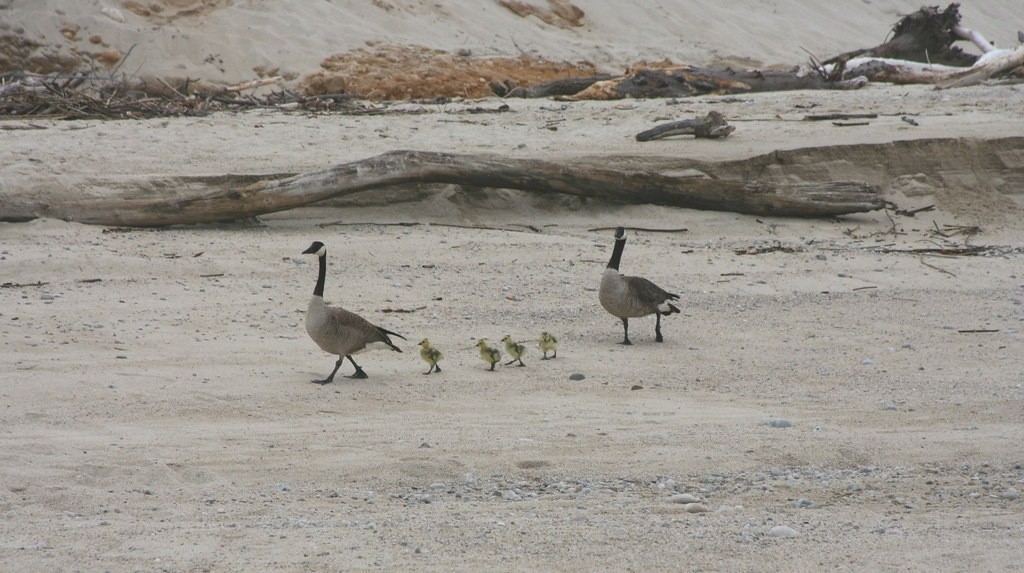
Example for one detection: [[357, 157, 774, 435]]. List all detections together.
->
[[539, 332, 558, 360], [300, 240, 408, 385], [599, 224, 681, 345], [417, 339, 444, 375], [502, 335, 528, 367]]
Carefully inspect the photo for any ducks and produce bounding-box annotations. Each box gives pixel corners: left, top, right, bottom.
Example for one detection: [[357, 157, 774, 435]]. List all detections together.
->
[[476, 340, 502, 371]]
